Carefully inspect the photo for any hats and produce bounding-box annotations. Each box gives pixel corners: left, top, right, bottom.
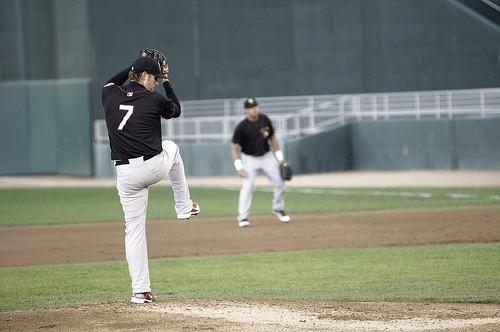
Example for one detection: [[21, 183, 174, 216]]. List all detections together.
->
[[244, 96, 258, 108], [130, 56, 164, 78]]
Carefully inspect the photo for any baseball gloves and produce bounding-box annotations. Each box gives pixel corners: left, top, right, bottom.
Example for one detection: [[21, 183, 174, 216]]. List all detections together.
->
[[139, 49, 169, 76], [279, 162, 293, 181]]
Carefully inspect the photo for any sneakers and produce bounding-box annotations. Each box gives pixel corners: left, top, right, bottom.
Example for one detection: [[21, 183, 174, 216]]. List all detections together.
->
[[130, 292, 156, 304], [271, 209, 291, 222], [177, 203, 200, 220], [237, 215, 251, 228]]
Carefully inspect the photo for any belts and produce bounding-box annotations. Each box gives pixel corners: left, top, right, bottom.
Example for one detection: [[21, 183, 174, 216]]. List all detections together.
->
[[116, 155, 155, 166]]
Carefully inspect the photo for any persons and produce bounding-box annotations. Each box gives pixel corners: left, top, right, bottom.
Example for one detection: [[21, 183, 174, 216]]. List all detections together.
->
[[102, 57, 200, 303], [231, 97, 291, 227]]
